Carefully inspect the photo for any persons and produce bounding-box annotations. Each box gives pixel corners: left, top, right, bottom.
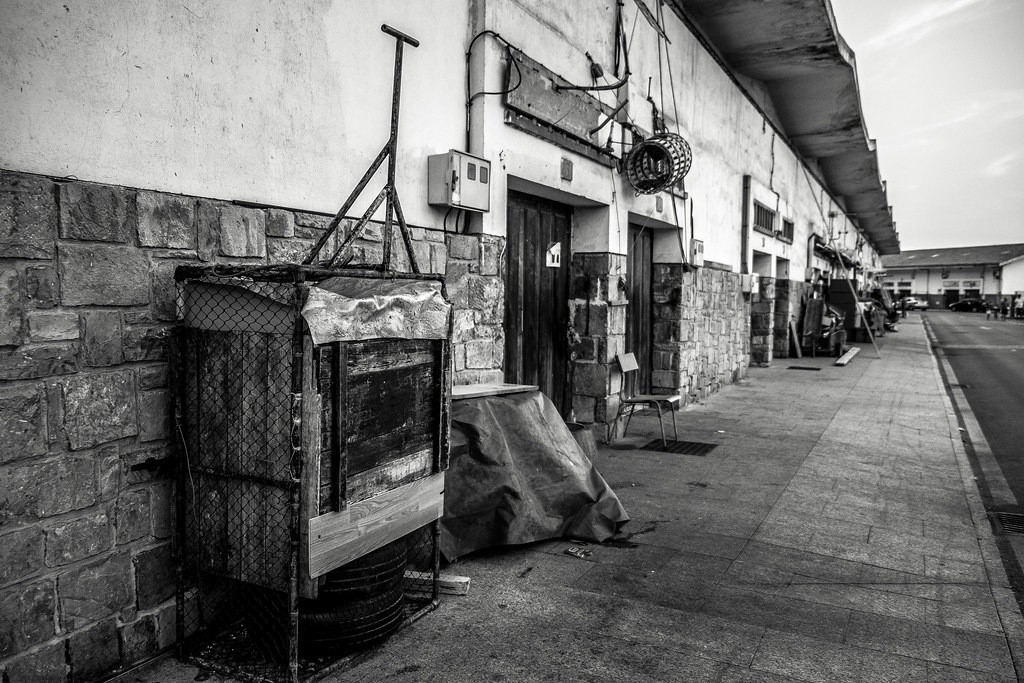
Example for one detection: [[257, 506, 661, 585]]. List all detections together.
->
[[988, 293, 1024, 320]]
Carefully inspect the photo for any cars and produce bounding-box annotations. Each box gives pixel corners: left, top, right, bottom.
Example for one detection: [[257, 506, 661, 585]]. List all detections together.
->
[[948, 297, 987, 313], [892, 295, 930, 311]]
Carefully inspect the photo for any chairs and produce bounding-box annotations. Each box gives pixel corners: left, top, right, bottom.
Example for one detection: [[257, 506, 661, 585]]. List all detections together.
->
[[605, 352, 683, 448]]
